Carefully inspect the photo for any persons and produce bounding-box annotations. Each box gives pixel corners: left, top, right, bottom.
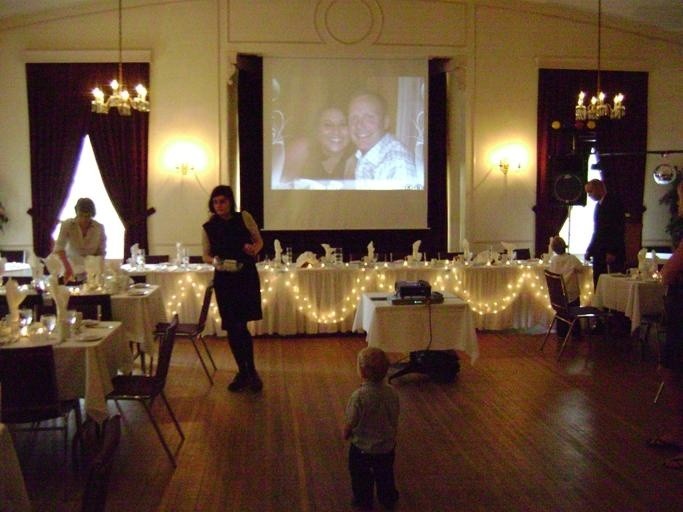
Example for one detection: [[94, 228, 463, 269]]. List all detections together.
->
[[347, 90, 415, 181], [51, 197, 107, 284], [584, 179, 627, 335], [547, 236, 585, 341], [200, 185, 264, 393], [342, 347, 400, 510], [648, 174, 683, 471], [280, 100, 358, 182]]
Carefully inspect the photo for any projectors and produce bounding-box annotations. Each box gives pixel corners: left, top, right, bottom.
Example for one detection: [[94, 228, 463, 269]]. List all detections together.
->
[[395, 280, 432, 297]]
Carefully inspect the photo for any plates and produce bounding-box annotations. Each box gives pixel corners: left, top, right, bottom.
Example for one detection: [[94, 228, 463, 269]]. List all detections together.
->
[[77, 335, 103, 340], [130, 290, 148, 295], [135, 283, 150, 288]]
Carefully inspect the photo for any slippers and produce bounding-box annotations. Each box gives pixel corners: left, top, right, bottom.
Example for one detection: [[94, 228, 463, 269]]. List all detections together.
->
[[646, 438, 682, 450], [664, 455, 683, 470]]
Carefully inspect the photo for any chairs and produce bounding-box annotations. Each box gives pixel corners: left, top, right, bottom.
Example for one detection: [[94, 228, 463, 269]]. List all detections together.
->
[[3, 345, 84, 502], [84, 313, 185, 468], [0, 321, 134, 462], [536, 266, 614, 365], [150, 280, 216, 385]]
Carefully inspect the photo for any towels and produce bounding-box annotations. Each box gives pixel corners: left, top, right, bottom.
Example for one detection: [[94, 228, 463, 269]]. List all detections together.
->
[[270, 239, 517, 264], [129, 243, 139, 260], [635, 246, 663, 272]]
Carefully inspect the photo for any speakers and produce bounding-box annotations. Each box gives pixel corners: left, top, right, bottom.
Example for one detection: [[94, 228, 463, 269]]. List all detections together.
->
[[547, 156, 588, 206]]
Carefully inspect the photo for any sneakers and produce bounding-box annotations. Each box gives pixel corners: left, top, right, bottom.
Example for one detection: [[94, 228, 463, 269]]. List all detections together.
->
[[227, 373, 249, 391], [381, 496, 398, 511], [573, 336, 583, 342], [351, 499, 373, 510], [558, 336, 568, 340], [247, 375, 264, 393]]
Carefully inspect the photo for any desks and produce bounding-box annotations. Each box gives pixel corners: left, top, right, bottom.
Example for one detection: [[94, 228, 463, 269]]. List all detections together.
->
[[351, 286, 475, 386], [117, 256, 596, 338], [0, 255, 165, 376]]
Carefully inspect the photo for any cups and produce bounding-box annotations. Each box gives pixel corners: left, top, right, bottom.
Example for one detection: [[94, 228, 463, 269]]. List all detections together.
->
[[0, 307, 83, 338]]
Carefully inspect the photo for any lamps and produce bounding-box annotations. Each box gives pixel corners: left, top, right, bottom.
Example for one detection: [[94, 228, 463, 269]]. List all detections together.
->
[[87, 1, 152, 118], [574, 1, 628, 126]]
